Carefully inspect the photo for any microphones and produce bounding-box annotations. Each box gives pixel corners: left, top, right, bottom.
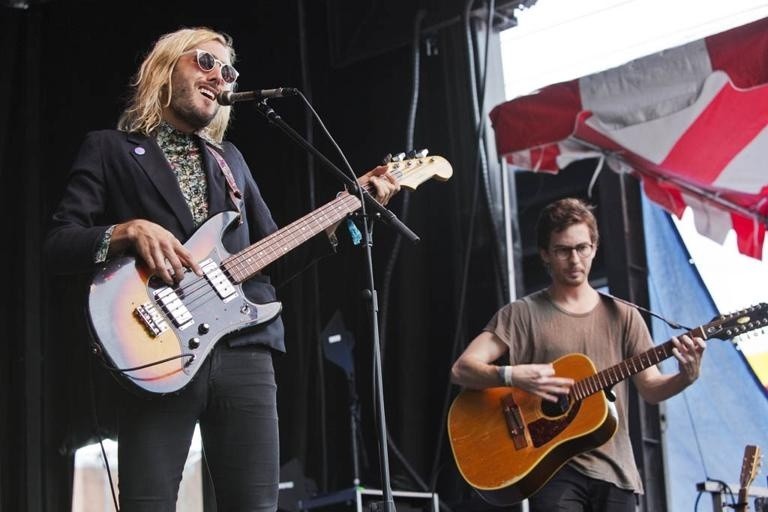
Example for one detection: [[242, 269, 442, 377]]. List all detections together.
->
[[216, 87, 297, 106]]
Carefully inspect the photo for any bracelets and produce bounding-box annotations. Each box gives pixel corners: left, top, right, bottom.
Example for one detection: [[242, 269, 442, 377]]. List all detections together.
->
[[505, 365, 513, 387]]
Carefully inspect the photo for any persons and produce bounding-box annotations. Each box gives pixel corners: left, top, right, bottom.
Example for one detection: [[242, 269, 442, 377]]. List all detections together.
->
[[449, 197, 707, 512], [43, 28, 401, 512]]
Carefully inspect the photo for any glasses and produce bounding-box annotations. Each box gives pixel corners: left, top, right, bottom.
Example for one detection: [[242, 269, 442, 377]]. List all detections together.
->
[[549, 242, 595, 261], [181, 49, 240, 85]]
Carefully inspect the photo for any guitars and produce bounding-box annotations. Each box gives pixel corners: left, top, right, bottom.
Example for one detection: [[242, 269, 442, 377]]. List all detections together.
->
[[733, 444, 763, 511], [446, 302, 768, 505], [81, 143, 455, 395]]
